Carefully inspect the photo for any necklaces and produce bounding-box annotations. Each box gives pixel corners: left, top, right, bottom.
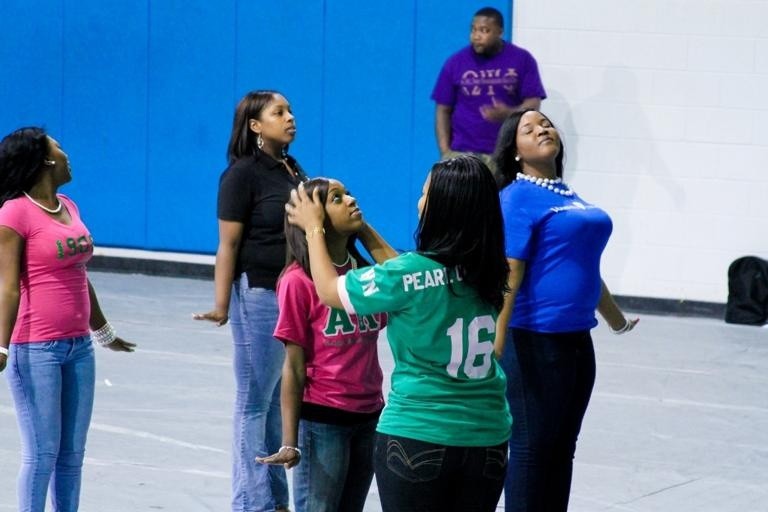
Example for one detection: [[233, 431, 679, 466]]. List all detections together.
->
[[331, 249, 351, 268], [515, 172, 574, 196]]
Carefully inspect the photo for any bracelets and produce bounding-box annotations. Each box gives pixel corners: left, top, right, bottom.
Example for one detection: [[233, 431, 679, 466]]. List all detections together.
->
[[609, 319, 631, 336], [91, 322, 117, 346], [0, 346, 9, 356], [277, 446, 302, 457]]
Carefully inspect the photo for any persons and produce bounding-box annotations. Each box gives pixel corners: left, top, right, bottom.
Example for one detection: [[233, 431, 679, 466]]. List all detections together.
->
[[487, 106, 639, 512], [431, 6, 546, 192], [254, 176, 385, 512], [0, 126, 136, 512], [22, 189, 61, 214], [284, 152, 512, 511], [190, 91, 311, 512]]
